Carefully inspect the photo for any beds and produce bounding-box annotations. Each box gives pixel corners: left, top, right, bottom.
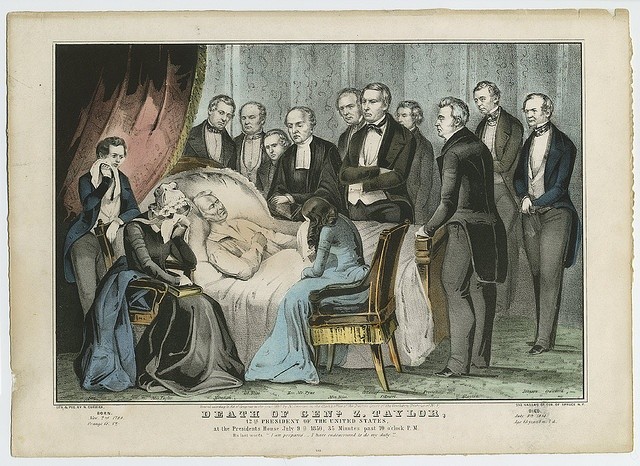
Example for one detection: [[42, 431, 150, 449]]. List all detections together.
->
[[128, 151, 451, 371]]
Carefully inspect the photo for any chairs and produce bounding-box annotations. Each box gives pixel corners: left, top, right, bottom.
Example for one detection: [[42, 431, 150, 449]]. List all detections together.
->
[[307, 219, 409, 393], [94, 217, 169, 328]]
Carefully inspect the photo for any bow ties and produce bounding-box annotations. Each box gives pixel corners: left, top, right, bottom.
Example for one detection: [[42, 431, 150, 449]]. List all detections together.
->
[[246, 134, 259, 140], [207, 128, 221, 133], [365, 118, 386, 136]]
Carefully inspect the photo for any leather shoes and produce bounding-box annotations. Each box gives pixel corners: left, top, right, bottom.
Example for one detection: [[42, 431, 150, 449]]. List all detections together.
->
[[528, 345, 548, 355], [526, 341, 535, 346], [435, 368, 454, 377]]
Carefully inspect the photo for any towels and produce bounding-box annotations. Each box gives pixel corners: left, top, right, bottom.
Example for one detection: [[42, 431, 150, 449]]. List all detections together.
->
[[297, 221, 308, 259], [90, 158, 121, 199], [160, 213, 189, 242]]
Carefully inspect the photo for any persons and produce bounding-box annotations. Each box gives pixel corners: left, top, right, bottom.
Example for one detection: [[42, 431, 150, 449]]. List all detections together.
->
[[191, 190, 298, 281], [64, 136, 140, 322], [231, 101, 273, 192], [414, 97, 508, 379], [334, 87, 382, 164], [396, 100, 432, 229], [338, 83, 415, 231], [178, 94, 237, 172], [470, 80, 525, 316], [513, 93, 580, 357], [255, 129, 291, 190], [122, 182, 245, 396], [245, 196, 372, 384], [266, 106, 342, 219]]
